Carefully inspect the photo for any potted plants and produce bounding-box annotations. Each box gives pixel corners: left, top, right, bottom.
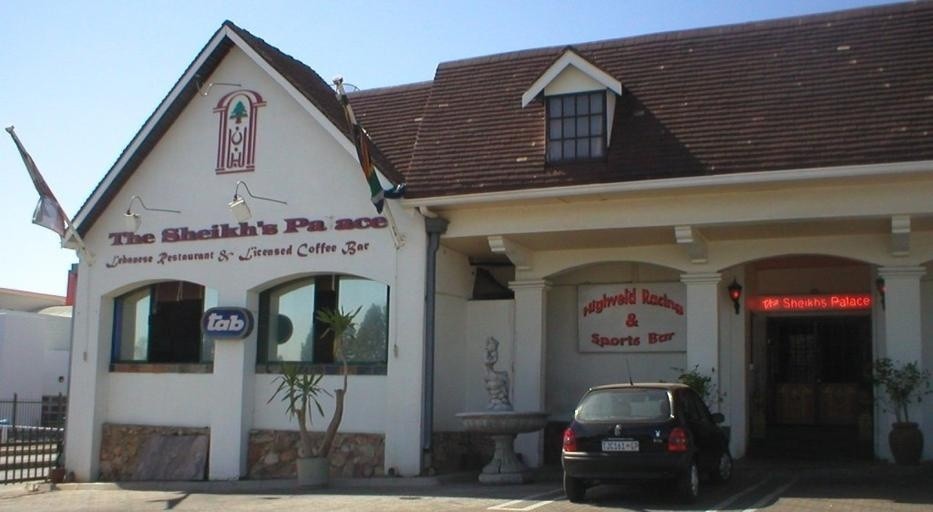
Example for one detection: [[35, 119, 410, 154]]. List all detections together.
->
[[875, 359, 932, 466], [262, 302, 366, 489]]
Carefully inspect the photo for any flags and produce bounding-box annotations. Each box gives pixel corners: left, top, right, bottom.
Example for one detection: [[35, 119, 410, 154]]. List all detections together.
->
[[7, 127, 65, 239], [337, 84, 407, 215]]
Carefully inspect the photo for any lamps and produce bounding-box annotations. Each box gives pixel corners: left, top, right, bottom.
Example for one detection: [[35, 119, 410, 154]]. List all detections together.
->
[[120, 180, 288, 233], [876, 275, 887, 312], [727, 277, 742, 318]]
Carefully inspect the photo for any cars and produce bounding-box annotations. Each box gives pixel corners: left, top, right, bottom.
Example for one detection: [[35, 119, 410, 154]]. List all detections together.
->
[[561, 383, 733, 503]]
